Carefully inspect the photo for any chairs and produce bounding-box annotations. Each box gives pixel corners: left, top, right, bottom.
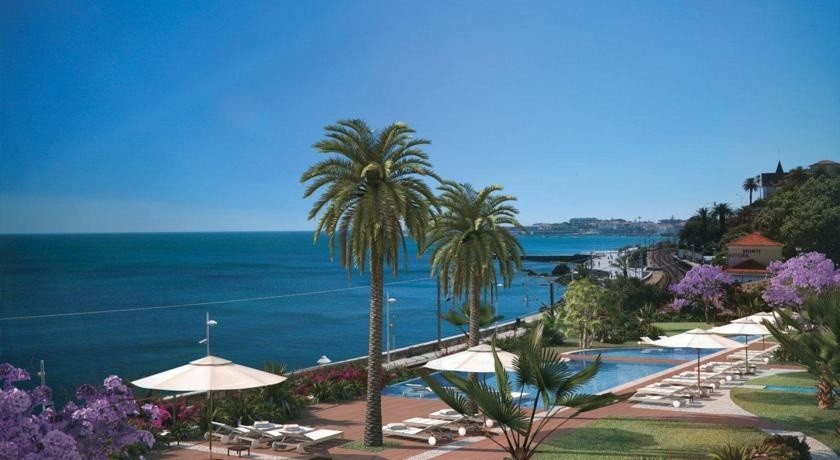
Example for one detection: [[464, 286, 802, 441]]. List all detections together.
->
[[628, 349, 772, 409], [205, 419, 344, 455], [382, 383, 498, 446]]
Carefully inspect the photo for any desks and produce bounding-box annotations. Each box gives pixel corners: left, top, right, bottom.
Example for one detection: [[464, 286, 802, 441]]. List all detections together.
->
[[227, 446, 250, 457]]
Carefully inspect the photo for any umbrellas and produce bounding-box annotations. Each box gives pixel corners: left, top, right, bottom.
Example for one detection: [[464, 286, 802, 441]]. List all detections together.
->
[[130, 355, 288, 460], [639, 307, 814, 400], [421, 343, 520, 422]]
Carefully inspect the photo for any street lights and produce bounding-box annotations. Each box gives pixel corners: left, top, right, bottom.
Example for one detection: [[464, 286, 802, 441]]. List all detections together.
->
[[199, 313, 217, 428], [496, 284, 503, 335], [386, 291, 397, 362]]
